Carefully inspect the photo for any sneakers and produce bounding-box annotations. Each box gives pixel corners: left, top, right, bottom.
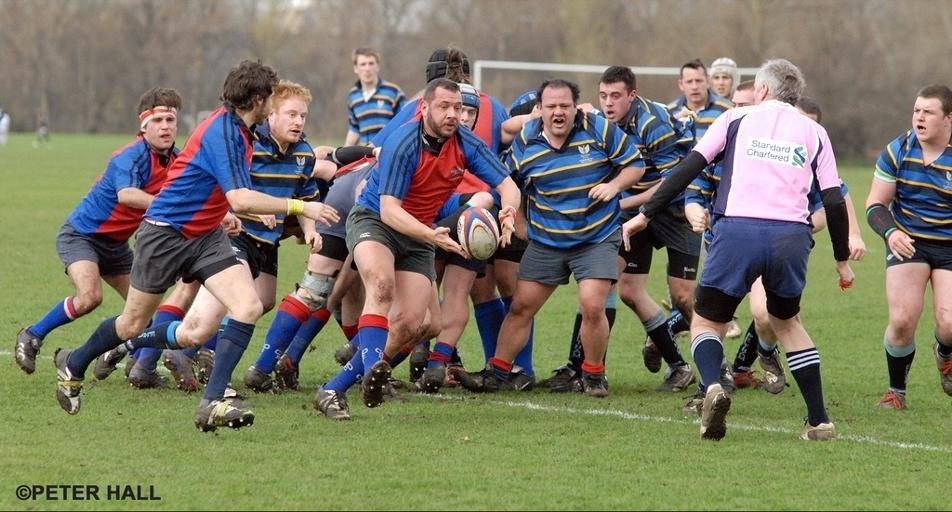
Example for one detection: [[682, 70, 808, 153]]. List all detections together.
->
[[15, 328, 42, 373], [657, 365, 695, 393], [546, 366, 577, 391], [196, 348, 219, 384], [275, 353, 302, 393], [504, 370, 536, 393], [163, 348, 201, 393], [573, 373, 608, 391], [876, 393, 907, 413], [732, 370, 760, 388], [933, 342, 951, 395], [583, 368, 607, 398], [453, 366, 504, 393], [758, 351, 787, 394], [682, 367, 736, 416], [390, 375, 402, 388], [125, 358, 134, 377], [444, 355, 465, 388], [92, 348, 122, 380], [361, 359, 391, 408], [54, 348, 85, 416], [128, 362, 168, 389], [799, 419, 836, 442], [408, 354, 426, 381], [642, 330, 663, 374], [334, 342, 365, 385], [701, 383, 732, 441], [243, 364, 281, 397], [312, 382, 350, 421], [194, 399, 255, 433], [416, 364, 446, 394], [222, 386, 241, 400]]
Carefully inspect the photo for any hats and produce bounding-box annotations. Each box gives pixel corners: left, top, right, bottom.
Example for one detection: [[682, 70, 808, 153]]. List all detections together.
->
[[709, 56, 742, 97]]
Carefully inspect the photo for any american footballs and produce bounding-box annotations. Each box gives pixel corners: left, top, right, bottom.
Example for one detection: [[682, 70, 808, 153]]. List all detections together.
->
[[456, 206, 501, 261]]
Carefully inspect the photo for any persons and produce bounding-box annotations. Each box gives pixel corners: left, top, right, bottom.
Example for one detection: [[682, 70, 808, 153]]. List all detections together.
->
[[865, 85, 952, 410]]
